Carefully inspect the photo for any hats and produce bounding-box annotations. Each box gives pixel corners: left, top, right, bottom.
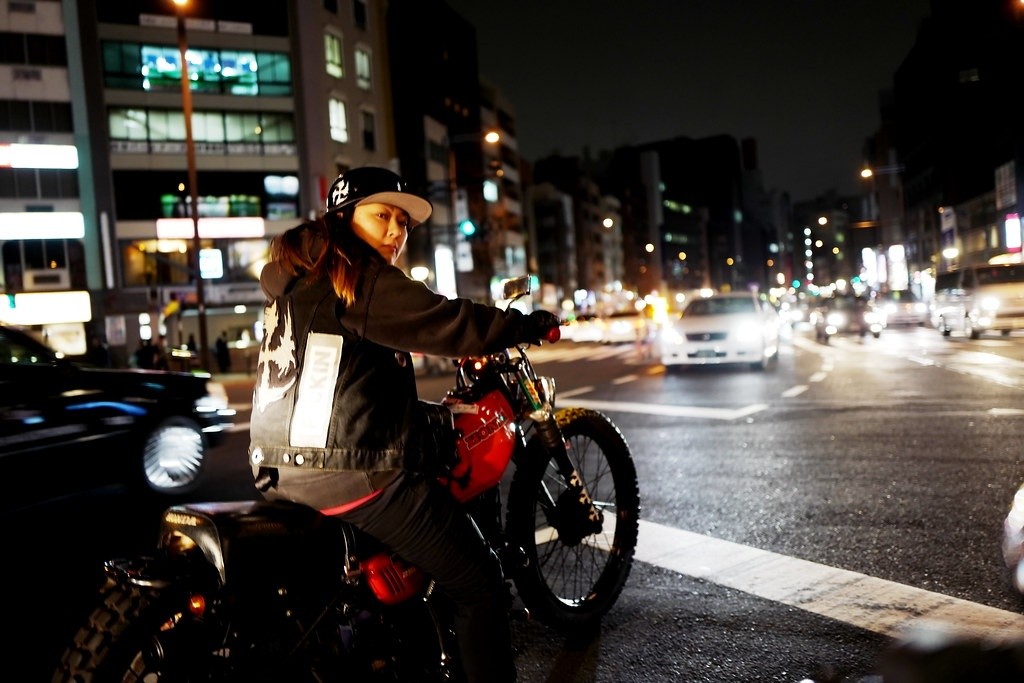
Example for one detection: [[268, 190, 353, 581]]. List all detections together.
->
[[325, 168, 432, 226]]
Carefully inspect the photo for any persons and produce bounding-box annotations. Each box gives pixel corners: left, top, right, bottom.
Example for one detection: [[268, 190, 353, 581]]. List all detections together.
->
[[134, 330, 231, 373], [247, 166, 561, 683]]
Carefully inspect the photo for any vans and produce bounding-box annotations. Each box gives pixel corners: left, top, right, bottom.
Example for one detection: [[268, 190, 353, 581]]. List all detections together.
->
[[931, 261, 1024, 341]]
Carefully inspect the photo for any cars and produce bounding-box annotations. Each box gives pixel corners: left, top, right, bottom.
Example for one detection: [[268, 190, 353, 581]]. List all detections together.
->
[[789, 308, 810, 330], [559, 309, 646, 344], [865, 288, 928, 328], [811, 293, 884, 341], [666, 291, 783, 374], [0, 322, 239, 499]]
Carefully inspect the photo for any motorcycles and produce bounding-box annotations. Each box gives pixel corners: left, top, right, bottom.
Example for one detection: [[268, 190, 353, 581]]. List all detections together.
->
[[44, 273, 642, 683]]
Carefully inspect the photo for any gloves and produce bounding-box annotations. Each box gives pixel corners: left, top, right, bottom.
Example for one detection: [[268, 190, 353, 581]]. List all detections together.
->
[[525, 309, 562, 347]]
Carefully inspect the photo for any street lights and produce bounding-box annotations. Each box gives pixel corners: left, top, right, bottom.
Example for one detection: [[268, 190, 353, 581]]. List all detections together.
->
[[172, 0, 212, 373]]
